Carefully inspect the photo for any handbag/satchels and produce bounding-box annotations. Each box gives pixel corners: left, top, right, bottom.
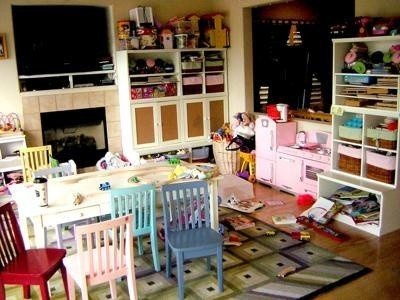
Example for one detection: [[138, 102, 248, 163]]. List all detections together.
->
[[233, 135, 255, 153]]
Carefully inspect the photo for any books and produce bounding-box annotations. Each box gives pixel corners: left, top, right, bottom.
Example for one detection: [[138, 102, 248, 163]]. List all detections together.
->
[[330, 186, 380, 225], [272, 213, 297, 224], [224, 214, 256, 231]]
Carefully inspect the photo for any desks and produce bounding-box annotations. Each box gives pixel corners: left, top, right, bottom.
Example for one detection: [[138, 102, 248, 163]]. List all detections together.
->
[[7, 159, 224, 297]]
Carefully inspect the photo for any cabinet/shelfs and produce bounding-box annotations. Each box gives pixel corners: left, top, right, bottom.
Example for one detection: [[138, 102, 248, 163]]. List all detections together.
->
[[180, 48, 228, 149], [317, 35, 400, 238], [0, 129, 27, 173], [255, 116, 331, 197], [116, 48, 180, 159]]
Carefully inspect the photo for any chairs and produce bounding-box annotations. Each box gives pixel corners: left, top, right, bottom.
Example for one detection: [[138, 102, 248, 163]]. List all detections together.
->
[[103, 182, 160, 272], [19, 144, 52, 183], [62, 213, 138, 300], [32, 165, 91, 249], [162, 182, 225, 300], [0, 202, 67, 300]]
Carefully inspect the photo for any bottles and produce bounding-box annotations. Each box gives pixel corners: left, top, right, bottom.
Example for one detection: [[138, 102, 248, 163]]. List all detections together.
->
[[116, 19, 159, 50]]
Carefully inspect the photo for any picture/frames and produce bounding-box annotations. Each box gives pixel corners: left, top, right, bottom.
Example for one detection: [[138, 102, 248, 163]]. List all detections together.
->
[[0, 31, 9, 60]]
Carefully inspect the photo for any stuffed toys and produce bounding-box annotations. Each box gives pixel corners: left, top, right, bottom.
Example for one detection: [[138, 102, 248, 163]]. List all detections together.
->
[[211, 112, 257, 182]]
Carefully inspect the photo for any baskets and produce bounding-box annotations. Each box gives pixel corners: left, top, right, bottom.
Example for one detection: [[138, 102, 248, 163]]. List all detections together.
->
[[213, 134, 240, 174], [338, 144, 361, 176], [365, 149, 396, 184], [367, 126, 397, 149], [339, 125, 362, 144]]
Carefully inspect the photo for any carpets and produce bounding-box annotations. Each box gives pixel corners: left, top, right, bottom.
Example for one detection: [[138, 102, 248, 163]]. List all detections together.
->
[[0, 192, 374, 300]]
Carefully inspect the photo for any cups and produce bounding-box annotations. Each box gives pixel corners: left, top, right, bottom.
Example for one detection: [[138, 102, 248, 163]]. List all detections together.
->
[[174, 34, 189, 49], [160, 34, 174, 49], [32, 177, 49, 208]]
